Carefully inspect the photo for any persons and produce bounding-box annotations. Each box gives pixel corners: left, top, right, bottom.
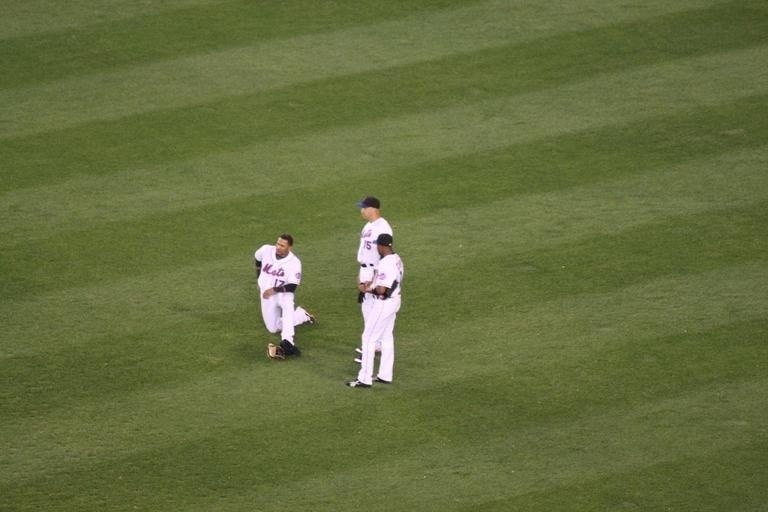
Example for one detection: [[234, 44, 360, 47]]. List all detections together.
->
[[344, 195, 405, 388], [254, 234, 317, 359]]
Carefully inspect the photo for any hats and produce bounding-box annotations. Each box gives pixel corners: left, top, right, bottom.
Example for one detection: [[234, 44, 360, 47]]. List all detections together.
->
[[372, 234, 394, 247], [357, 197, 381, 208]]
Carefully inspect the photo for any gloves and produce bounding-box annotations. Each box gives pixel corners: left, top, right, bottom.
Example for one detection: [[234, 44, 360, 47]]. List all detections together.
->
[[358, 291, 365, 303]]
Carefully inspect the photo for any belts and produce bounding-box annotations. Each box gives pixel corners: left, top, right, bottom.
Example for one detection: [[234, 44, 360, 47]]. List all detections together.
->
[[360, 264, 374, 268]]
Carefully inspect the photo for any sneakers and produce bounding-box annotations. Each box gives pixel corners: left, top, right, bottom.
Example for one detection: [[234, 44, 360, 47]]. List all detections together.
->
[[296, 305, 317, 325], [347, 375, 390, 388], [354, 347, 362, 363]]
